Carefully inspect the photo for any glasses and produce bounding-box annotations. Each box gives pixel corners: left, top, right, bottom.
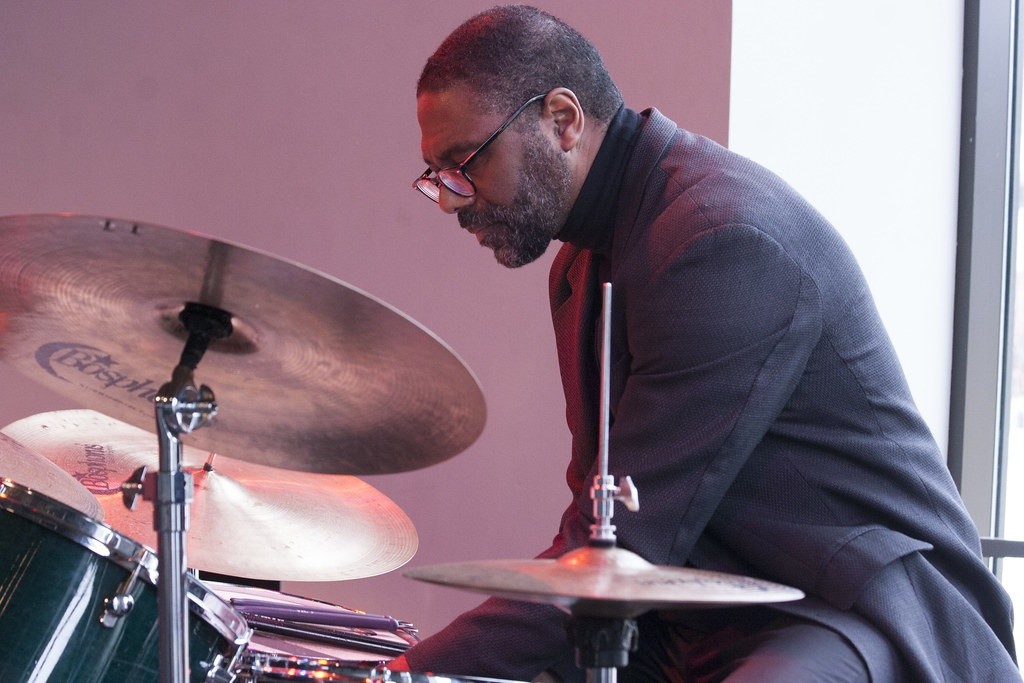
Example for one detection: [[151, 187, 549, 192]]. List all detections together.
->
[[411, 93, 586, 204]]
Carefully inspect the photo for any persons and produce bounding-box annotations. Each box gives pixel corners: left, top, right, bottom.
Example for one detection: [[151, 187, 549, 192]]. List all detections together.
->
[[396, 2, 1023, 683]]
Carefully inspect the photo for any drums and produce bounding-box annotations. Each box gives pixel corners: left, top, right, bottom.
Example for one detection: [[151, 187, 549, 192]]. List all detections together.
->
[[201, 581, 419, 683], [1, 481, 252, 683]]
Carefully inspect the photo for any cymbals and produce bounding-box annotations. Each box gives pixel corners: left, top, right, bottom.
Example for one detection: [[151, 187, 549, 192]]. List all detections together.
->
[[0, 210, 491, 478], [1, 405, 419, 583], [404, 548, 807, 617]]
